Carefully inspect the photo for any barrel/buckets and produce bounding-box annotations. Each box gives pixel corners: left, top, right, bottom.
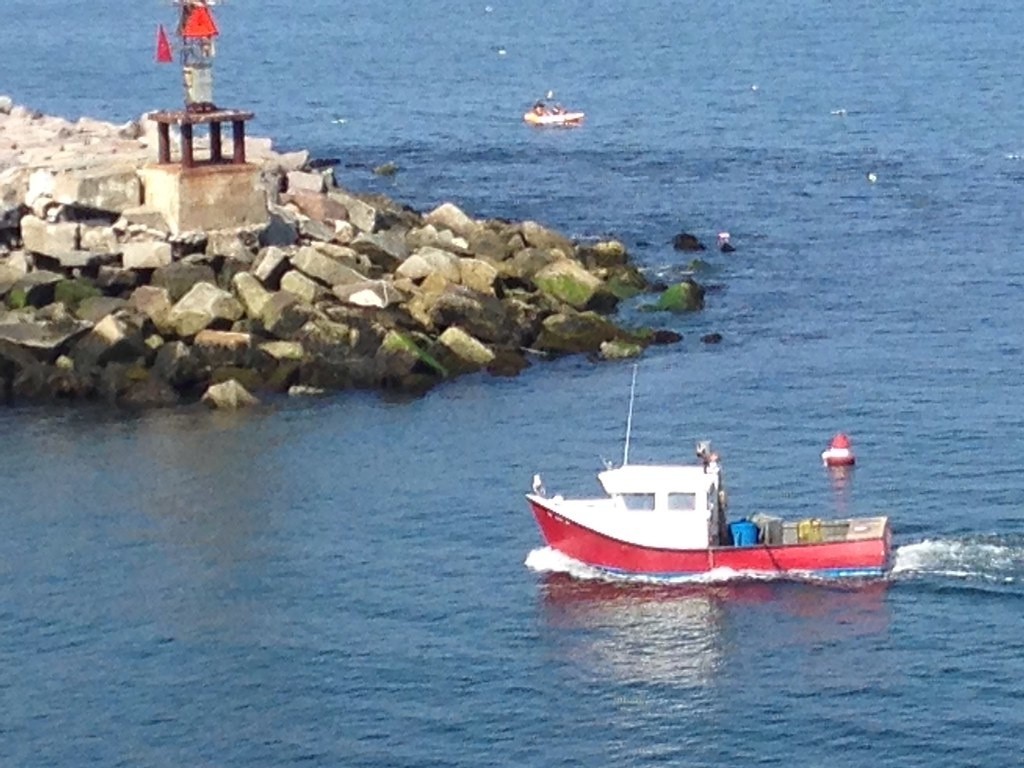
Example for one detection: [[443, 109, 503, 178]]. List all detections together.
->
[[730, 521, 757, 547]]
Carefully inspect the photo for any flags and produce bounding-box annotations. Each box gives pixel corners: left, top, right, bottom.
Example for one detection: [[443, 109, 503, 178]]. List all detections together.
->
[[157, 29, 173, 62]]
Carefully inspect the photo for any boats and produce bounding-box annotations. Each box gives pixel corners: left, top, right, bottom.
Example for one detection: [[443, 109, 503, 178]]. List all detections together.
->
[[523, 105, 586, 132], [524, 364, 892, 581]]
